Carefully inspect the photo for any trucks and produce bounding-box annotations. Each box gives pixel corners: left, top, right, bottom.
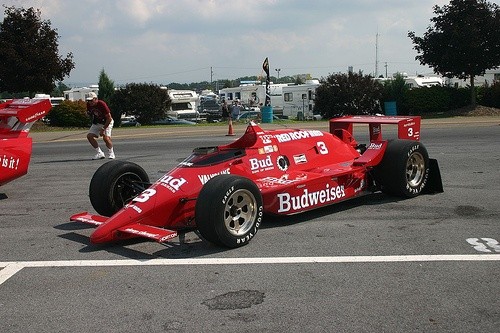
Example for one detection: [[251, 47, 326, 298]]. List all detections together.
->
[[30, 70, 448, 124]]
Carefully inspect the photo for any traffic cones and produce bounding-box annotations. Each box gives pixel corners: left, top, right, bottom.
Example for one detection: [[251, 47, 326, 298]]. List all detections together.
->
[[225, 117, 236, 136]]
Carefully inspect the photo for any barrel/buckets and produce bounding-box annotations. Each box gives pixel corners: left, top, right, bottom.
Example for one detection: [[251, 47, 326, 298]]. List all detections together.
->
[[385, 102, 397, 116], [262, 106, 273, 123]]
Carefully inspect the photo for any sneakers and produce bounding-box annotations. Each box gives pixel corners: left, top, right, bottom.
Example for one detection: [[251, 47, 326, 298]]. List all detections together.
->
[[92, 152, 105, 160], [108, 152, 115, 160]]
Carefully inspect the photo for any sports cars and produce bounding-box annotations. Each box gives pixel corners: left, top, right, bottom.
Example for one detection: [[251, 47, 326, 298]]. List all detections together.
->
[[69, 113, 446, 249], [0, 97, 53, 187]]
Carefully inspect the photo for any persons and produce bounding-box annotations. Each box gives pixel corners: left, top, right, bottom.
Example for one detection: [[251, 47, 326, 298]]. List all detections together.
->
[[222, 97, 242, 120], [84, 91, 115, 159]]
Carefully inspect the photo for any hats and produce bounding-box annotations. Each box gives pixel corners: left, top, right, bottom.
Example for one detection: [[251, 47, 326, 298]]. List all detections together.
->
[[86, 92, 97, 100]]
